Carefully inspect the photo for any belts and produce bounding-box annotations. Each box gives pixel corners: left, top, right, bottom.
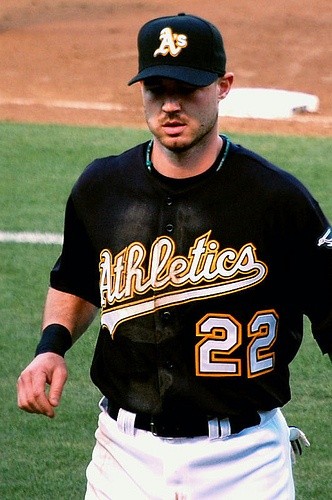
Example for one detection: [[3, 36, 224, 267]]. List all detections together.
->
[[99, 399, 262, 437]]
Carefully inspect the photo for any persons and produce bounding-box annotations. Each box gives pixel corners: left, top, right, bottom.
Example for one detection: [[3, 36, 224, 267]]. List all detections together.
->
[[18, 10, 332, 500]]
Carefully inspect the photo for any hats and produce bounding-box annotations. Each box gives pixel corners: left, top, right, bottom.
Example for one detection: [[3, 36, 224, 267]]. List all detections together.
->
[[127, 13, 225, 87]]
